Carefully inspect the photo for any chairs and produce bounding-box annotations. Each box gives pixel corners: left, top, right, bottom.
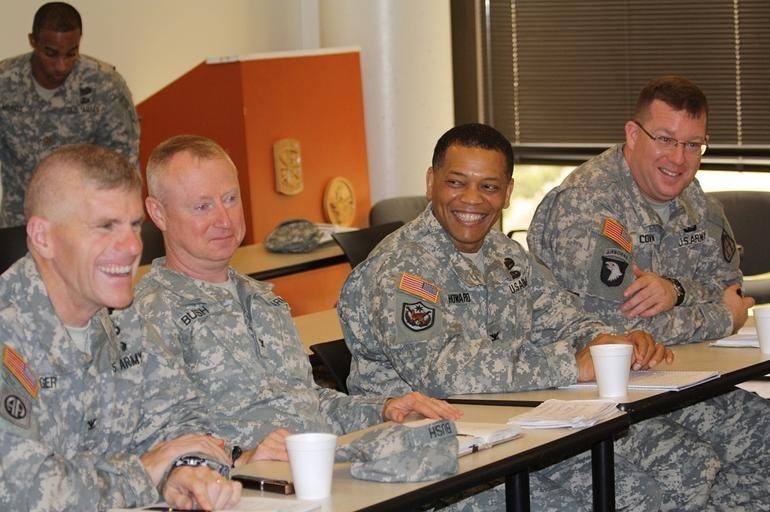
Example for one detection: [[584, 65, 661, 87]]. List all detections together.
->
[[702, 190, 770, 303], [308, 337, 353, 397], [331, 222, 406, 266], [369, 194, 529, 273]]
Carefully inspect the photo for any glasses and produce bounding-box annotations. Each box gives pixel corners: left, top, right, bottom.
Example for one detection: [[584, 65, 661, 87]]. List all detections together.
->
[[633, 121, 708, 156]]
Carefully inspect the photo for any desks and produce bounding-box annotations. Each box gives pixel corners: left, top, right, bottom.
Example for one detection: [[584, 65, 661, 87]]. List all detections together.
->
[[109, 403, 629, 512], [128, 223, 362, 364], [446, 315, 770, 512]]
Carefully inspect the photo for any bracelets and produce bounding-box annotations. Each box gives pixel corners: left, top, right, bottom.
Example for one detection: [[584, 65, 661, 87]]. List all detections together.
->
[[232, 446, 242, 467]]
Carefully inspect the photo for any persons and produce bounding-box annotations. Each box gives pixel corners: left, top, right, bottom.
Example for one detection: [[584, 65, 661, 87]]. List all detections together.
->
[[0, 143, 243, 512], [0, 2, 141, 229], [527, 77, 770, 511], [132, 135, 464, 468], [337, 123, 677, 512]]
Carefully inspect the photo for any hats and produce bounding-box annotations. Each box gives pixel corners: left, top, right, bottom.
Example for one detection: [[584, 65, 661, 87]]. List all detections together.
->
[[264, 220, 323, 254], [335, 419, 459, 483]]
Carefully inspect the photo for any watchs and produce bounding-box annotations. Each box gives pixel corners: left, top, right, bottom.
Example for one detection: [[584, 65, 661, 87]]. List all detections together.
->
[[668, 278, 686, 306], [175, 454, 207, 467]]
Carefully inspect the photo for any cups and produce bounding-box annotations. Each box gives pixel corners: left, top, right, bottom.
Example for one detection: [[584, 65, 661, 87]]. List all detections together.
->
[[589, 343, 634, 398], [285, 432, 337, 502], [752, 307, 770, 354]]
